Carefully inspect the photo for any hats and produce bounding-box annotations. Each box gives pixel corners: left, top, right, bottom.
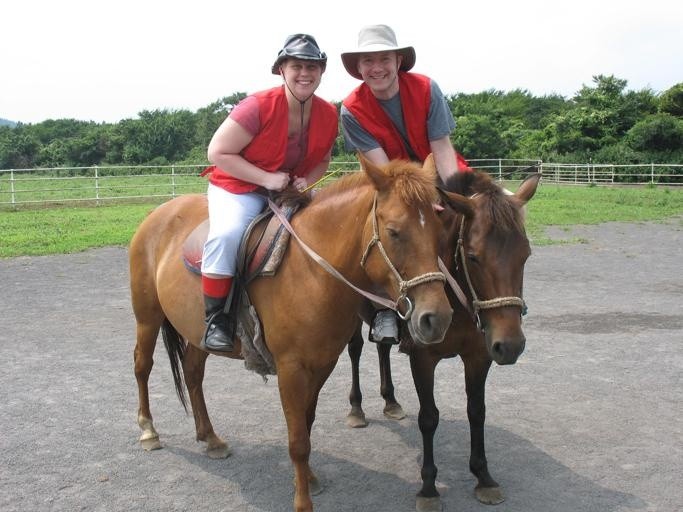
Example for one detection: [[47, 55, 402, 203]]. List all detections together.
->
[[272, 33, 327, 75], [341, 24, 416, 80]]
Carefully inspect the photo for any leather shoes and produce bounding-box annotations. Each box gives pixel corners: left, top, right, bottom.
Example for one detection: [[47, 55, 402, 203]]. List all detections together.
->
[[203, 318, 236, 351]]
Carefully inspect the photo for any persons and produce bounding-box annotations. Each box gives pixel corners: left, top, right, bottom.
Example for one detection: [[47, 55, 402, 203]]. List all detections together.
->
[[198, 32, 339, 352], [340, 24, 526, 344]]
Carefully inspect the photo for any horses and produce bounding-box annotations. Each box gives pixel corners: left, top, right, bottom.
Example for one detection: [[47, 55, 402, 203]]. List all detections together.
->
[[127, 145, 456, 512], [343, 166, 545, 512]]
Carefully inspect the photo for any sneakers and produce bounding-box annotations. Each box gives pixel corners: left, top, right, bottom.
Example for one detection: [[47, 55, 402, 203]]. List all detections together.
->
[[373, 308, 400, 344]]
